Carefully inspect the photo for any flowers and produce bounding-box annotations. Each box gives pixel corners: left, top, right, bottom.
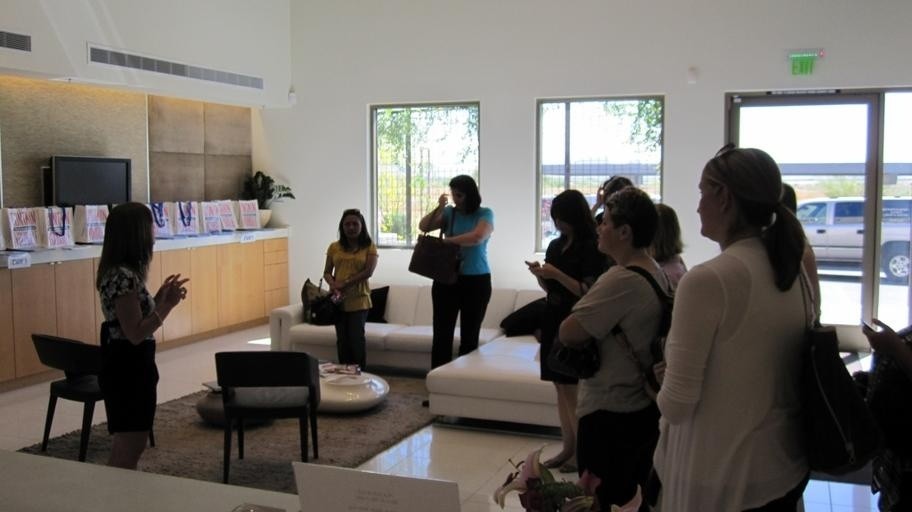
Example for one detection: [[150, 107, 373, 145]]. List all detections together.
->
[[485, 441, 648, 511]]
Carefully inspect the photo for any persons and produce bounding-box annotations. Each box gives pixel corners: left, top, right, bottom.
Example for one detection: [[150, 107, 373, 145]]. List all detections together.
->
[[323, 206, 383, 374], [409, 175, 496, 396], [92, 202, 186, 469], [652, 146, 820, 510], [590, 175, 634, 225], [860, 317, 912, 512], [641, 194, 689, 299], [768, 177, 821, 323], [524, 186, 602, 478], [559, 187, 673, 512]]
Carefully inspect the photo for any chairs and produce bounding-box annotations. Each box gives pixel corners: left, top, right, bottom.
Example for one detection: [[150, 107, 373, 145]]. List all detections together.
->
[[211, 350, 322, 486], [26, 330, 158, 469]]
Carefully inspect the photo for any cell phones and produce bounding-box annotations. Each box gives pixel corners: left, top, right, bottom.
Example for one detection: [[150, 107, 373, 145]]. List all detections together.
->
[[860, 319, 877, 332], [525, 260, 536, 270], [330, 291, 344, 304]]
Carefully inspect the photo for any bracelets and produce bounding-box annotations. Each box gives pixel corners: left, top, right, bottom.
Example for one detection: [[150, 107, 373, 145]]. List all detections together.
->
[[151, 310, 165, 327]]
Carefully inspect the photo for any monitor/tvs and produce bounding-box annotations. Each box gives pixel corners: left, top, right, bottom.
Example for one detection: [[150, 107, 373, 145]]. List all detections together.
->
[[42, 167, 52, 207], [52, 154, 132, 206]]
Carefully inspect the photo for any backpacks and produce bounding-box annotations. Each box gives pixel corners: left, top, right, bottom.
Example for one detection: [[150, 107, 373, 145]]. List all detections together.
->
[[610, 266, 675, 403]]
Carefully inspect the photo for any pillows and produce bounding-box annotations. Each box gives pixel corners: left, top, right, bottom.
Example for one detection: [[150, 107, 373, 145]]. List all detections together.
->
[[301, 278, 329, 324], [500, 297, 548, 338], [366, 285, 389, 323]]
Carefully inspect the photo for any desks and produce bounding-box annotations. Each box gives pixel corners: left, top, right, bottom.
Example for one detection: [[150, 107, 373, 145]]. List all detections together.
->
[[0, 445, 303, 511]]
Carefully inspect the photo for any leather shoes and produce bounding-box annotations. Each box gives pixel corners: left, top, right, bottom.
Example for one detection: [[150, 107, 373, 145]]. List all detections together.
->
[[543, 456, 569, 468], [560, 461, 578, 473]]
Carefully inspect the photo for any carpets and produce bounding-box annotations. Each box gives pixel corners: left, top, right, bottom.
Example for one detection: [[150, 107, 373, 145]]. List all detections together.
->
[[17, 374, 427, 499]]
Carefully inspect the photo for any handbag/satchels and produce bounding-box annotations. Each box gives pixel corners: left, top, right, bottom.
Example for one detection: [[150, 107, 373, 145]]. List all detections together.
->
[[409, 204, 462, 283], [211, 198, 238, 231], [170, 201, 199, 236], [546, 334, 601, 380], [232, 198, 261, 230], [74, 204, 110, 244], [0, 207, 42, 251], [796, 318, 876, 477], [309, 276, 342, 326], [199, 201, 222, 234], [145, 201, 173, 239], [40, 206, 74, 250]]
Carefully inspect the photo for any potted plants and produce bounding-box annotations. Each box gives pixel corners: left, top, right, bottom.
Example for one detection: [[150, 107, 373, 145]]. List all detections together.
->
[[239, 169, 299, 228]]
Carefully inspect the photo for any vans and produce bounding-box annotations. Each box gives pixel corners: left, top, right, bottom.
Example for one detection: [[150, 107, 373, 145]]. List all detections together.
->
[[796, 196, 912, 282]]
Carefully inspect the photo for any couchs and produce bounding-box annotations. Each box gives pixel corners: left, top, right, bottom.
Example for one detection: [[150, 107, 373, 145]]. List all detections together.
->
[[268, 284, 568, 443]]
